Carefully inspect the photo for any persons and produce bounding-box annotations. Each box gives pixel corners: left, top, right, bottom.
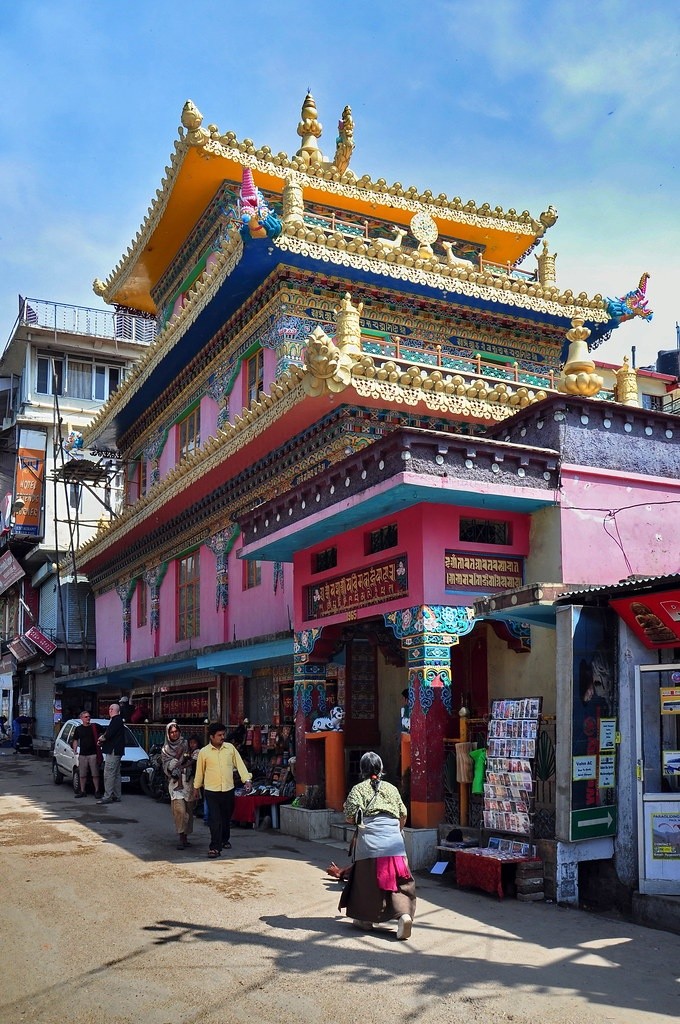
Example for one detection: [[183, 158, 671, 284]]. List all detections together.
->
[[399, 687, 410, 732], [338, 751, 417, 941], [160, 721, 201, 850], [96, 703, 126, 805], [193, 721, 253, 859], [631, 602, 679, 644], [12, 715, 36, 755], [71, 710, 104, 799]]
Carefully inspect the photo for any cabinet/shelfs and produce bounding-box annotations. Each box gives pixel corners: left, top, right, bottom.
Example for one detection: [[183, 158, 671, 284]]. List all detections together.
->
[[480, 697, 543, 856]]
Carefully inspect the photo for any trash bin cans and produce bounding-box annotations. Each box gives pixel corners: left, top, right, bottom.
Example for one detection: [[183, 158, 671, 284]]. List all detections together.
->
[[13, 715, 35, 748]]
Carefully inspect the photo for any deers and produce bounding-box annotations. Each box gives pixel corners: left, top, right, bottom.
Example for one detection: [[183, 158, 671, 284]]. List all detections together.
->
[[442, 241, 475, 271], [372, 225, 407, 249]]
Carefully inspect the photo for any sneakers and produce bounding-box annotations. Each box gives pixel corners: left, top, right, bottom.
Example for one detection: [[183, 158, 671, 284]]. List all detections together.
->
[[353, 919, 373, 930], [397, 914, 412, 939]]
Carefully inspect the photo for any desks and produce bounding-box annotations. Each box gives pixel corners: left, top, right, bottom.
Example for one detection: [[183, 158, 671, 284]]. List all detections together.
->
[[230, 796, 288, 829]]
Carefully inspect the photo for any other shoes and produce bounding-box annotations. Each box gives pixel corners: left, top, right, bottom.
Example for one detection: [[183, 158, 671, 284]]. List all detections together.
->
[[223, 841, 233, 849], [208, 850, 221, 857], [111, 795, 120, 802], [74, 791, 88, 799], [96, 797, 113, 804], [94, 792, 102, 798], [176, 842, 183, 851]]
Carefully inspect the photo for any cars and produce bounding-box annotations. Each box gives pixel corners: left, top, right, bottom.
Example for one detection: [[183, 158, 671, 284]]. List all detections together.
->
[[51, 718, 151, 795]]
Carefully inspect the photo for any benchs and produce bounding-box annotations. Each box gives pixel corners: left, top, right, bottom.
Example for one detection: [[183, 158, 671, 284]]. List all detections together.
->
[[435, 846, 544, 901]]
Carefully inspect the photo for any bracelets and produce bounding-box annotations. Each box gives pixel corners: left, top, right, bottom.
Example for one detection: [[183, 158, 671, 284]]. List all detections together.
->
[[246, 780, 253, 783]]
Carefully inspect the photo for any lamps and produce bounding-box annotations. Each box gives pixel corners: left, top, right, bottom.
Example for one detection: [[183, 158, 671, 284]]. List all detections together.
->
[[12, 494, 25, 513]]
